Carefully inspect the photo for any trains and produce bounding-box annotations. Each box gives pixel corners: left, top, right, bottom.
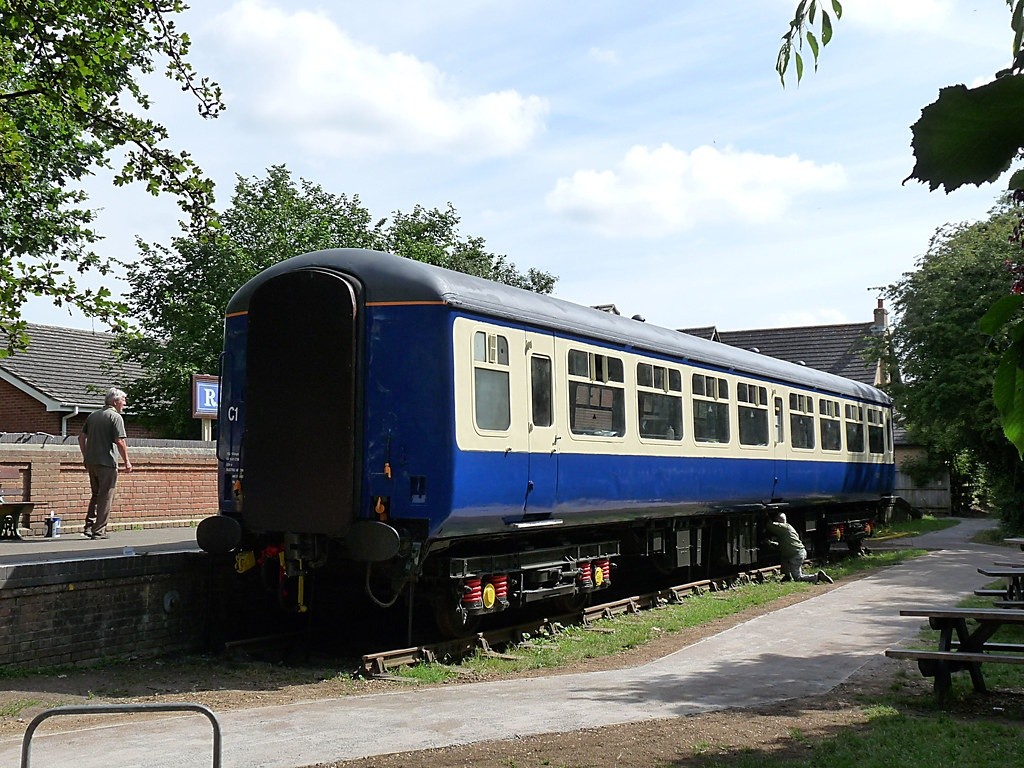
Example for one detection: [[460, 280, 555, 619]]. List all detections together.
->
[[196, 247, 899, 678]]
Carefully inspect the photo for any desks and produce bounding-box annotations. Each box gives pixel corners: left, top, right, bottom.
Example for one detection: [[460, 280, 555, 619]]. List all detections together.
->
[[976, 567, 1024, 611], [1004, 537, 1024, 551], [901, 606, 1024, 703], [993, 561, 1024, 566]]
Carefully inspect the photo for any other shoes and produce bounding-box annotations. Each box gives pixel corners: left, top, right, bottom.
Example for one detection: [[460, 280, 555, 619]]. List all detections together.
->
[[818, 570, 834, 584], [91, 532, 110, 540], [84, 526, 92, 537], [780, 576, 792, 582]]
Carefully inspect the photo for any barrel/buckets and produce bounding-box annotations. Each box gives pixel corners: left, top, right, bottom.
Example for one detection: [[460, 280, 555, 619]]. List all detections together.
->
[[43, 517, 62, 537]]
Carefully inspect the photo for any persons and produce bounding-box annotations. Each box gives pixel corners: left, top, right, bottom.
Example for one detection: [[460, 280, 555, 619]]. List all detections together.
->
[[78, 388, 132, 540], [764, 511, 833, 584]]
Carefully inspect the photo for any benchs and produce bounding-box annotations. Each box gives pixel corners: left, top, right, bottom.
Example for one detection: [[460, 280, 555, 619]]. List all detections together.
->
[[0, 468, 49, 541], [885, 640, 1024, 672], [974, 590, 1024, 608]]
